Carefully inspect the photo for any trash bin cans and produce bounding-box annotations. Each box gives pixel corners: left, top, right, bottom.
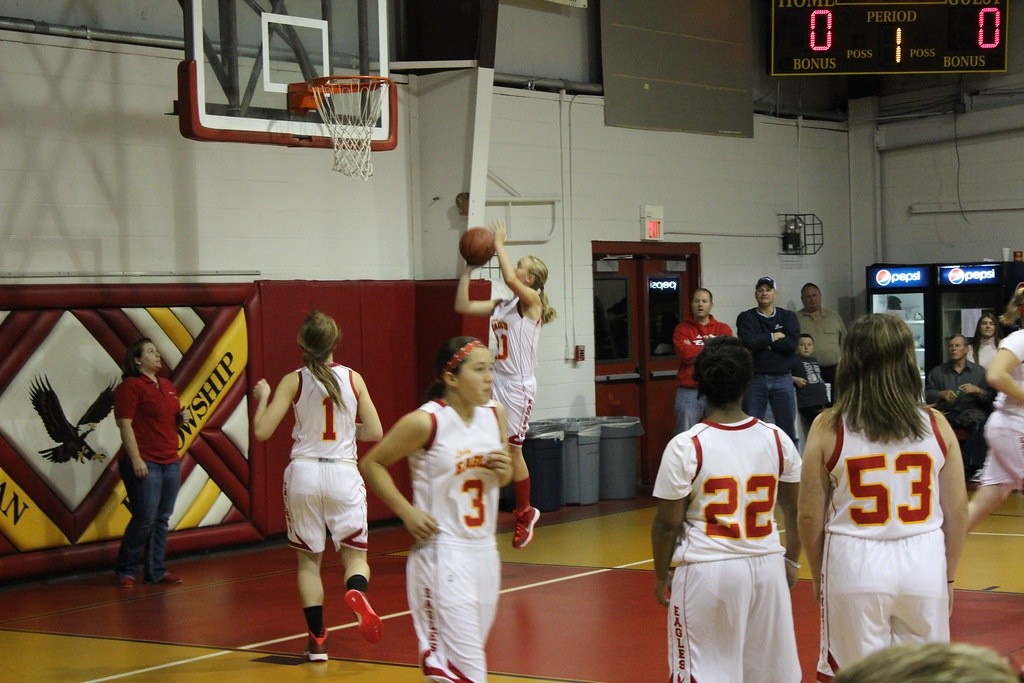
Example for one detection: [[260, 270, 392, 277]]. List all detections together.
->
[[538, 417, 600, 505], [597, 416, 642, 499], [504, 423, 564, 512]]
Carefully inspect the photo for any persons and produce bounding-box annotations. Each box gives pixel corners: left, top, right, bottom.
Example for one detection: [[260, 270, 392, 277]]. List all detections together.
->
[[114, 338, 190, 588], [965, 313, 1004, 369], [830, 643, 1024, 683], [924, 334, 996, 482], [736, 277, 800, 456], [795, 283, 847, 405], [673, 287, 733, 434], [359, 336, 512, 683], [796, 315, 970, 683], [792, 333, 832, 426], [968, 282, 1024, 533], [254, 312, 383, 660], [454, 219, 558, 548], [650, 336, 802, 683]]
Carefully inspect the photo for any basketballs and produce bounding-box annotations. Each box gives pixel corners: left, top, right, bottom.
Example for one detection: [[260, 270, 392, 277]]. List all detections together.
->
[[460, 228, 496, 267]]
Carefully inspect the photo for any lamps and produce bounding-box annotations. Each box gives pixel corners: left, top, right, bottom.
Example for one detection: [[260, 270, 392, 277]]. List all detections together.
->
[[782, 220, 805, 251]]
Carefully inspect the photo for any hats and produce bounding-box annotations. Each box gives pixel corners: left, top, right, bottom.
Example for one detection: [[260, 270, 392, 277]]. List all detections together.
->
[[757, 277, 776, 290]]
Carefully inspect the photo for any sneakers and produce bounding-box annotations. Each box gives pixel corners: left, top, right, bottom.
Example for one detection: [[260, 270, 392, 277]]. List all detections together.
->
[[304, 627, 329, 662], [116, 576, 134, 588], [158, 572, 184, 585], [345, 589, 384, 644], [512, 505, 540, 549]]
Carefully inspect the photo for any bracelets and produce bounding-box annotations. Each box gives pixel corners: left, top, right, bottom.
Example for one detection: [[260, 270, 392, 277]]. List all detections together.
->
[[784, 557, 801, 569]]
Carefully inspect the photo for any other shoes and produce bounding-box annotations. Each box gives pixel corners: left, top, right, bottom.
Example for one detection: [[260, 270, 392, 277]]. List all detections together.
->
[[968, 469, 984, 482]]
